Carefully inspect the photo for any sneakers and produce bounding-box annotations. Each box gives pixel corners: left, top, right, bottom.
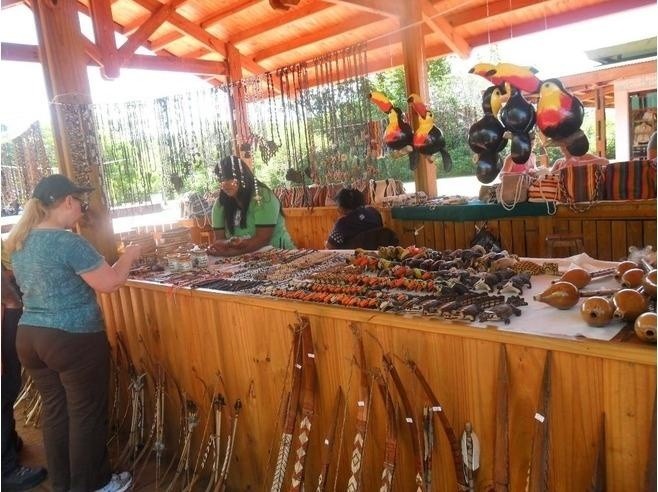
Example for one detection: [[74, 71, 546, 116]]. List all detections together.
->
[[96, 471, 133, 492], [1, 464, 48, 492]]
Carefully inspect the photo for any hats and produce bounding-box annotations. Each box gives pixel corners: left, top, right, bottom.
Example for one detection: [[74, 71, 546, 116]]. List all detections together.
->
[[31, 174, 95, 205]]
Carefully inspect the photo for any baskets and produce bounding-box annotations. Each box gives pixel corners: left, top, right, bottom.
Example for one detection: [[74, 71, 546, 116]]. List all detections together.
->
[[122, 225, 194, 264]]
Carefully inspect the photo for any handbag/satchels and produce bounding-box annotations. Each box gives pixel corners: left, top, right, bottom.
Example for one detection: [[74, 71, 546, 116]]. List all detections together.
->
[[500, 158, 657, 204]]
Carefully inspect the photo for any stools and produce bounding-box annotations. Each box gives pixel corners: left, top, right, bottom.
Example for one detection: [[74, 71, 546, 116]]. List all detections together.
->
[[545, 233, 584, 259]]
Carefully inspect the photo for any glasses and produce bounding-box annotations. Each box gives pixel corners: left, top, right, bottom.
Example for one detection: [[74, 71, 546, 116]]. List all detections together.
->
[[72, 197, 90, 213]]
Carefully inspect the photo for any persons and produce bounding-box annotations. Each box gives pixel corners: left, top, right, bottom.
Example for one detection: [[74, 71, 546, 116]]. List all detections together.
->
[[1, 239, 48, 492], [498, 133, 537, 181], [551, 129, 609, 175], [1, 174, 142, 492], [323, 187, 399, 250], [647, 131, 657, 161], [205, 155, 295, 257]]
